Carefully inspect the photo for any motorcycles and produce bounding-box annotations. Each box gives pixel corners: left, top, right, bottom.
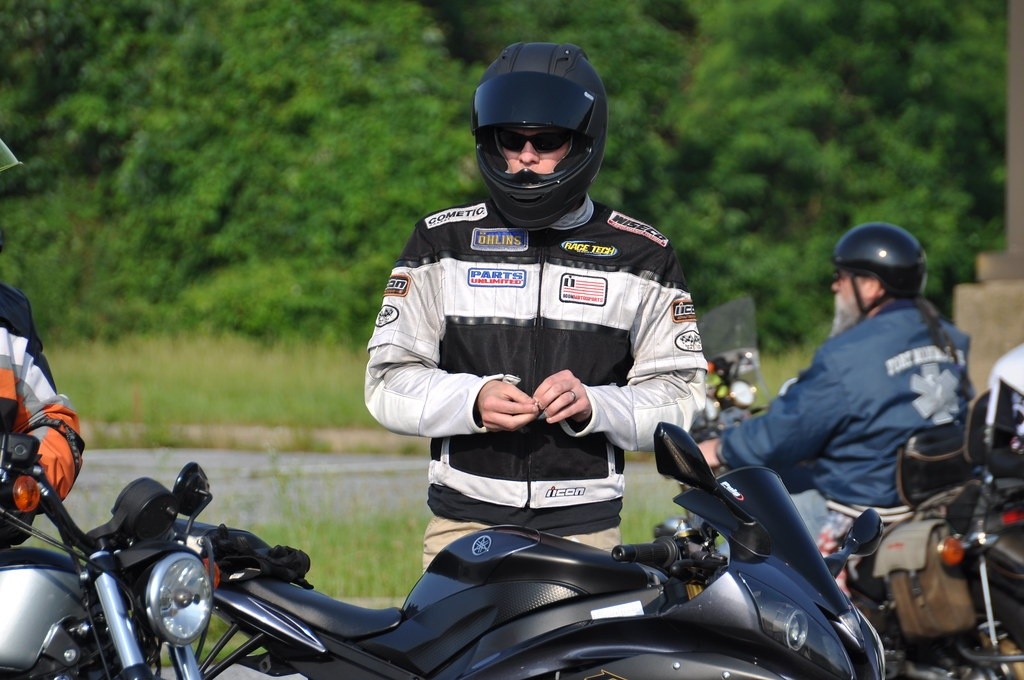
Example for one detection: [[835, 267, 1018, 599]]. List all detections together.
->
[[0, 422, 886, 680], [687, 295, 1024, 680]]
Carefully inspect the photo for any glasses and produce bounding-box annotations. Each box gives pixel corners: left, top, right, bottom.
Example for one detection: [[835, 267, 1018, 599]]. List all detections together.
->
[[832, 270, 852, 281], [496, 126, 572, 153]]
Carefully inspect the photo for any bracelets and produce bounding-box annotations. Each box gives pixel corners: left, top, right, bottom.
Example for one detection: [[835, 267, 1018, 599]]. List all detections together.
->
[[717, 443, 727, 465]]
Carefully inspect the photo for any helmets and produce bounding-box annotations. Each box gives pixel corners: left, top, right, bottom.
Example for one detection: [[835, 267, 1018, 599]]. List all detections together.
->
[[470, 42, 608, 231], [831, 222, 928, 297]]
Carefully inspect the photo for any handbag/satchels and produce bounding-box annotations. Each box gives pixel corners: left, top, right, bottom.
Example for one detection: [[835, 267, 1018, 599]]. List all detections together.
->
[[868, 485, 977, 642]]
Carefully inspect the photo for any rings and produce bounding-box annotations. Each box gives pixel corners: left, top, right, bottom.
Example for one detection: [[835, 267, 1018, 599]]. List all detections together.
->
[[569, 391, 577, 402]]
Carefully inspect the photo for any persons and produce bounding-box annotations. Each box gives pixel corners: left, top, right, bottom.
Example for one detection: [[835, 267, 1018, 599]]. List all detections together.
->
[[697, 222, 980, 565], [366, 42, 707, 576], [0, 139, 84, 549]]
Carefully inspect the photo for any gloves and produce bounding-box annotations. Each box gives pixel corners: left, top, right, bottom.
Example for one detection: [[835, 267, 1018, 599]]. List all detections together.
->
[[200, 525, 315, 590], [0, 480, 39, 546]]
